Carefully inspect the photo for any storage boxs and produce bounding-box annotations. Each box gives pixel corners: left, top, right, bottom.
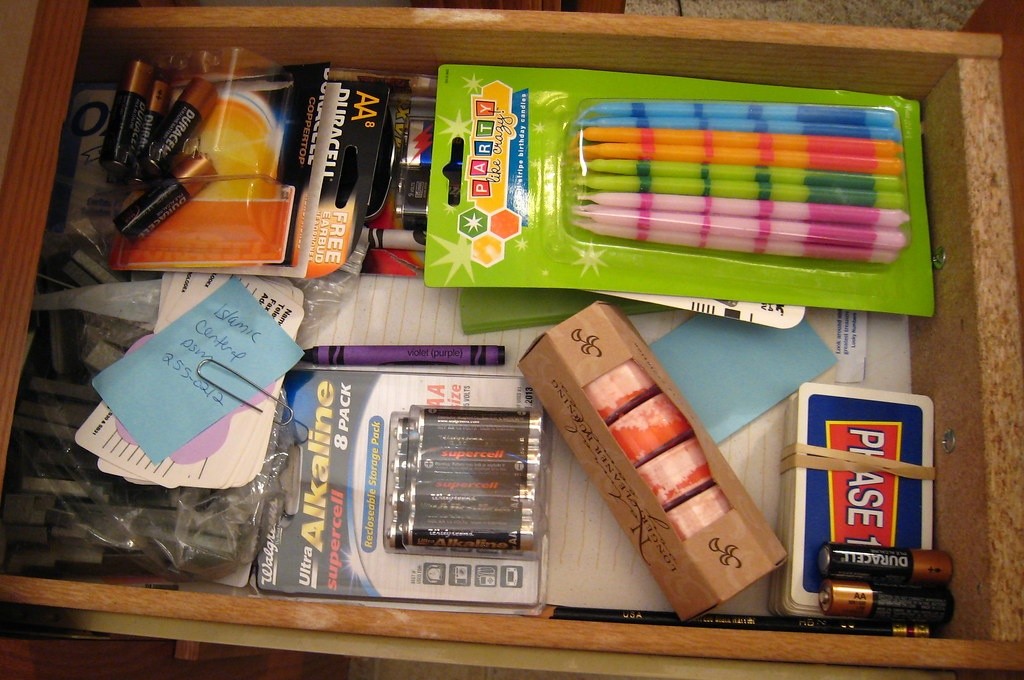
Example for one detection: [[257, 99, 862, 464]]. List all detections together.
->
[[519, 302, 790, 622]]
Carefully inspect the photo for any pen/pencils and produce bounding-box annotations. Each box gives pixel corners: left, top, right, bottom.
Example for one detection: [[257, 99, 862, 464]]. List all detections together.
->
[[362, 227, 424, 249], [299, 343, 504, 370]]
